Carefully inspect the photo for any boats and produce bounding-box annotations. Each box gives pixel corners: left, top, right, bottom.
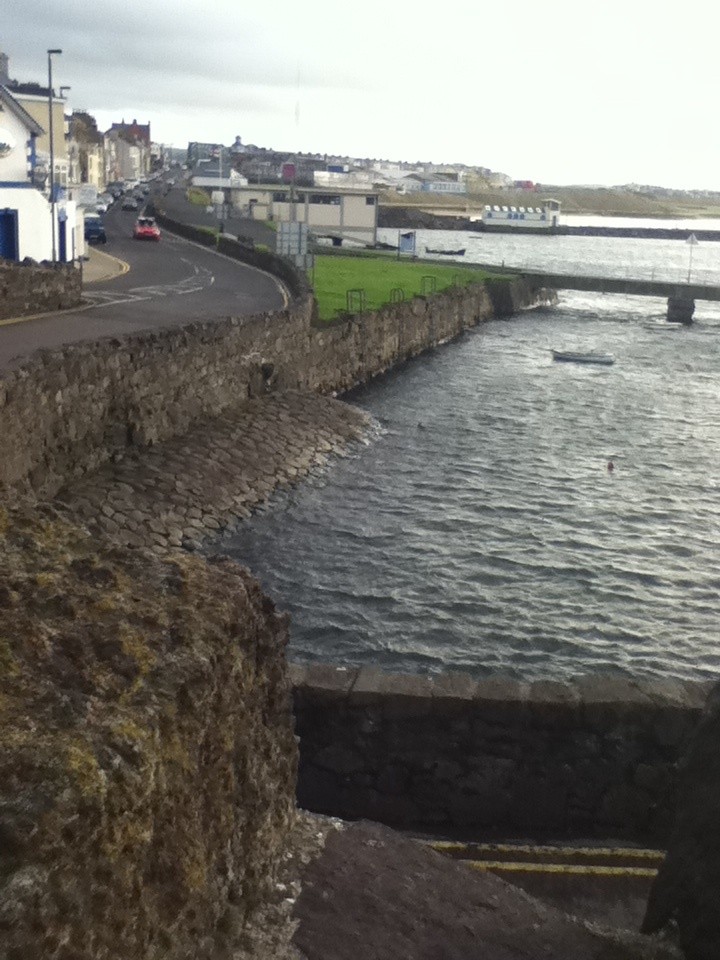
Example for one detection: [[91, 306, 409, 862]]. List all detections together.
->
[[482, 198, 562, 228], [424, 246, 467, 257], [550, 348, 618, 367]]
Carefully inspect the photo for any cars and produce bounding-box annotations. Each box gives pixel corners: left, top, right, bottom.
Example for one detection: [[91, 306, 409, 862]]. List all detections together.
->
[[95, 165, 174, 215], [133, 215, 163, 239], [132, 189, 146, 202], [122, 196, 139, 211], [83, 212, 107, 244]]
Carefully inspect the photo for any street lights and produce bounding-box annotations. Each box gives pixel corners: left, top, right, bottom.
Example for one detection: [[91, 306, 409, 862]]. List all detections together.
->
[[47, 50, 63, 262]]
